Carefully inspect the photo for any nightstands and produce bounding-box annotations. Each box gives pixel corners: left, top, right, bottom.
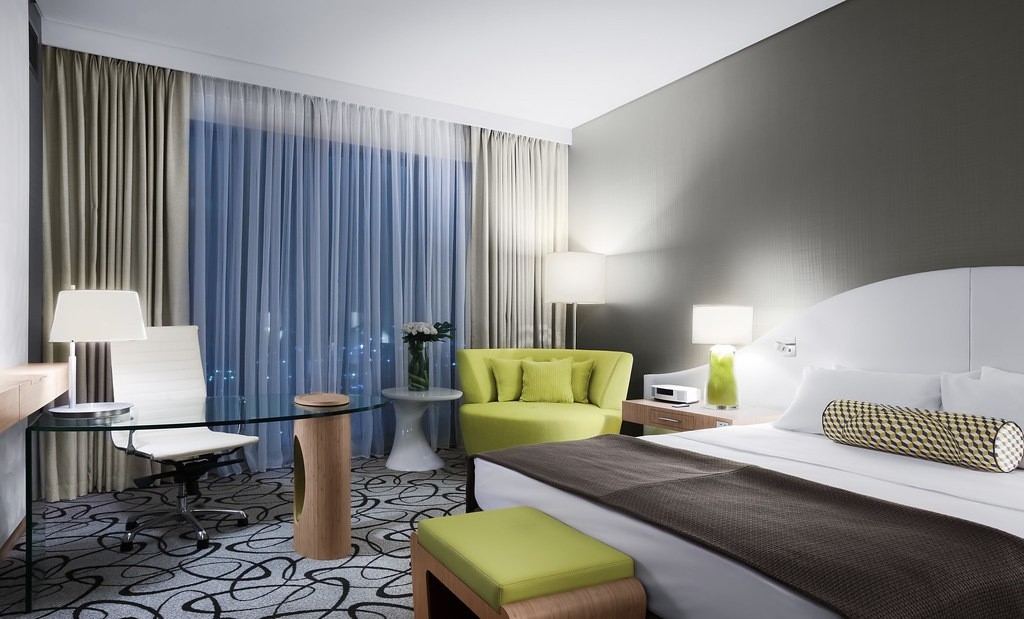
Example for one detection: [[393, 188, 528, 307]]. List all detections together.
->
[[622, 397, 783, 436]]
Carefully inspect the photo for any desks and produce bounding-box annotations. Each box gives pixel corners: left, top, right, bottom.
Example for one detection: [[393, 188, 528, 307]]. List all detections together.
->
[[23, 393, 388, 612]]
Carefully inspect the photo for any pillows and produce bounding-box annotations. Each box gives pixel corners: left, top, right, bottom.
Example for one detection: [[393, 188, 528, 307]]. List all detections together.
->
[[840, 410, 961, 467], [844, 368, 942, 411], [941, 372, 1024, 469], [489, 356, 533, 402], [821, 399, 1024, 473], [979, 367, 1024, 385], [519, 356, 574, 404], [771, 364, 847, 435], [550, 357, 595, 404]]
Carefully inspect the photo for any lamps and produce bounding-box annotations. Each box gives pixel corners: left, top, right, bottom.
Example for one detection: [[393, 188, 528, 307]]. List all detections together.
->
[[49, 284, 146, 419], [692, 304, 753, 410], [544, 252, 608, 350]]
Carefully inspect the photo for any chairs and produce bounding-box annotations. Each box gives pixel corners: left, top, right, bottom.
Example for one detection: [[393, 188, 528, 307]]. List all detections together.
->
[[110, 324, 259, 553]]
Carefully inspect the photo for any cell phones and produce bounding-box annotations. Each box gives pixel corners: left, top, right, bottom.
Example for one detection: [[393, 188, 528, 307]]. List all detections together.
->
[[672, 404, 689, 408]]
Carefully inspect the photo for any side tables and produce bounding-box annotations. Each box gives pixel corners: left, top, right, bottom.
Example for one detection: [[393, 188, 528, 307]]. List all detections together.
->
[[382, 386, 463, 471]]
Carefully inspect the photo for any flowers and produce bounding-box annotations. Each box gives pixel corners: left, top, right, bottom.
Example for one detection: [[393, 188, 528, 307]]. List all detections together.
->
[[401, 319, 456, 389]]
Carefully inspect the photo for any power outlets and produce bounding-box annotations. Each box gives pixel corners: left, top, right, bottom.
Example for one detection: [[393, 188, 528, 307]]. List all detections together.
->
[[777, 335, 796, 357], [716, 420, 728, 428]]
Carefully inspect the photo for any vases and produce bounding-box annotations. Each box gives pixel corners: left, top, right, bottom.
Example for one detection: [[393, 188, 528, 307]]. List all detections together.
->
[[406, 347, 430, 391]]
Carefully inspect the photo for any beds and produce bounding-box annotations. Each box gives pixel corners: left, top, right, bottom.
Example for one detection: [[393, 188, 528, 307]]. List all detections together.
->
[[466, 265, 1024, 619]]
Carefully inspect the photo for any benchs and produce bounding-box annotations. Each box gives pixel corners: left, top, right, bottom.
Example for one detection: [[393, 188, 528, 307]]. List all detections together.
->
[[410, 505, 647, 619]]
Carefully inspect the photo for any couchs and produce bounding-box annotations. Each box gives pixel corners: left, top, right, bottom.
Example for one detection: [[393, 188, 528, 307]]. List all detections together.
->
[[454, 348, 634, 457]]
